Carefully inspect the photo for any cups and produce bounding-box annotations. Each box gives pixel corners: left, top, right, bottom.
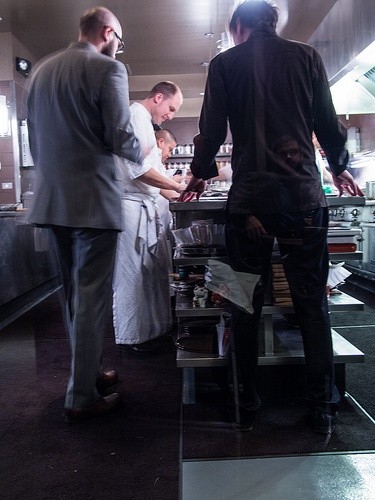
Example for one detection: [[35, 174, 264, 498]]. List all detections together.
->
[[216, 323, 231, 356]]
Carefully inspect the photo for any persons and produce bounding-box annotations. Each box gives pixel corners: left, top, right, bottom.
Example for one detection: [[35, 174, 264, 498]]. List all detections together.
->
[[112, 81, 187, 354], [25, 7, 155, 419], [155, 127, 232, 184], [177, 0, 368, 433]]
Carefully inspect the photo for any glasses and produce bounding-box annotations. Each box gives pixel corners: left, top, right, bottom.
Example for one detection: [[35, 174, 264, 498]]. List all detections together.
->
[[104, 25, 125, 52]]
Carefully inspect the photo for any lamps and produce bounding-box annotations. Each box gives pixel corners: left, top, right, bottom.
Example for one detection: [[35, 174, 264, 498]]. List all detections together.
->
[[205, 9, 215, 40]]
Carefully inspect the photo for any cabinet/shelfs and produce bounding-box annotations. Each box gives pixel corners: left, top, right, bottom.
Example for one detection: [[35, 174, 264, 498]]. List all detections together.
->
[[167, 195, 365, 408]]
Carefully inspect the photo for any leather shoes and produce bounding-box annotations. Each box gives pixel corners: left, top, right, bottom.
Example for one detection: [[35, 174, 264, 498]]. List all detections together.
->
[[97, 370, 118, 387], [118, 345, 153, 357], [65, 393, 121, 423], [314, 406, 339, 433], [232, 407, 256, 432]]
